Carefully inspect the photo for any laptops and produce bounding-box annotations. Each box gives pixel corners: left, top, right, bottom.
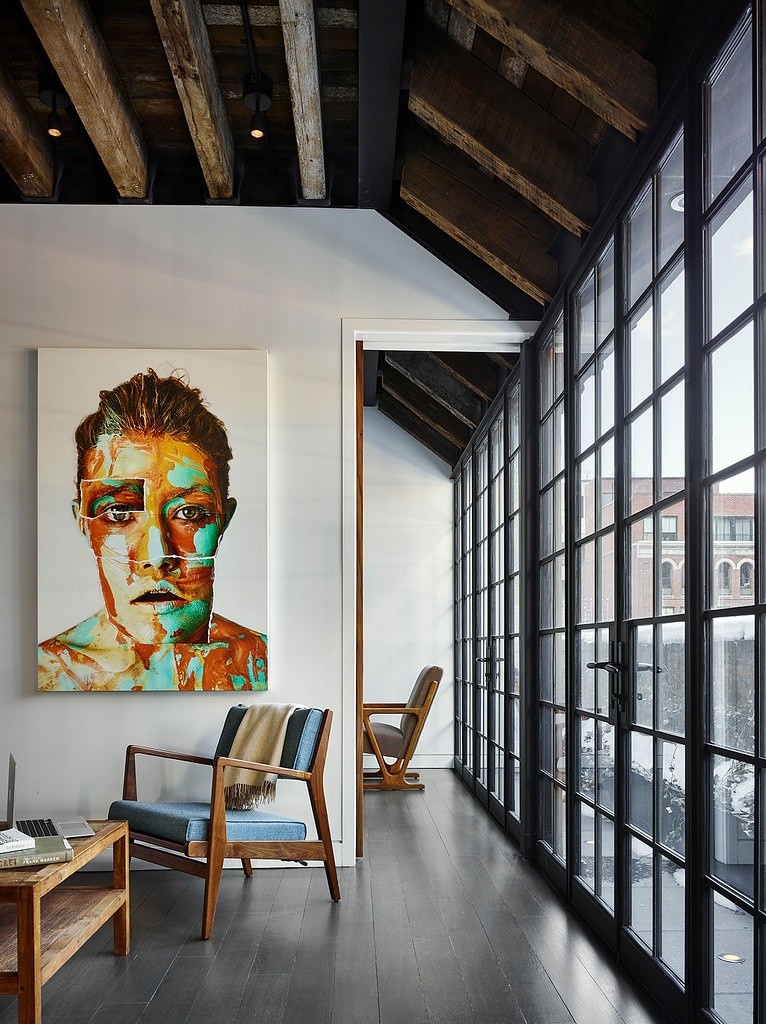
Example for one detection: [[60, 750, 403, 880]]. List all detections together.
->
[[8, 753, 96, 839]]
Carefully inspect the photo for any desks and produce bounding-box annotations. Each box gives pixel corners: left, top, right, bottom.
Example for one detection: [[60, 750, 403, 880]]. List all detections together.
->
[[0, 820, 131, 1024]]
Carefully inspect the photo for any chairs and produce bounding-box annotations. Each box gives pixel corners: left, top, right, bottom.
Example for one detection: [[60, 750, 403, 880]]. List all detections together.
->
[[108, 703, 341, 941], [363, 664, 444, 791]]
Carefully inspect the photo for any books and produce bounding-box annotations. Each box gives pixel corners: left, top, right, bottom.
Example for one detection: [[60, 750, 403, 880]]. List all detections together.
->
[[0, 828, 36, 854], [0, 835, 74, 870]]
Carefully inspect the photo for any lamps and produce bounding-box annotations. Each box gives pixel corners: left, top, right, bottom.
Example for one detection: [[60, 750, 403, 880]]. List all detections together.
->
[[46, 99, 63, 137], [250, 101, 265, 139]]
